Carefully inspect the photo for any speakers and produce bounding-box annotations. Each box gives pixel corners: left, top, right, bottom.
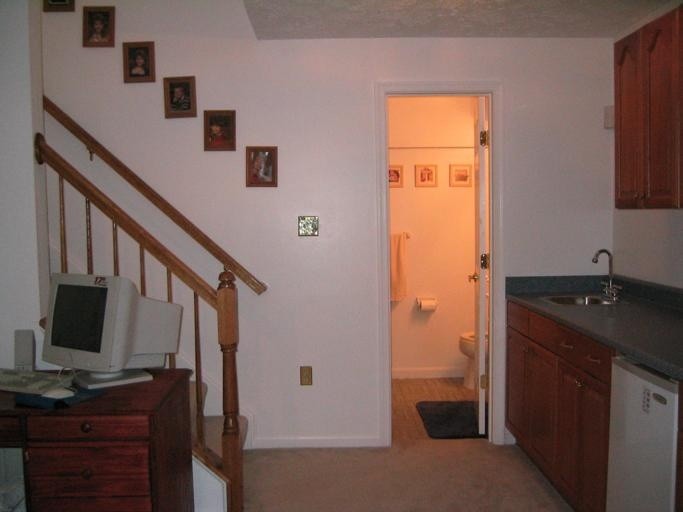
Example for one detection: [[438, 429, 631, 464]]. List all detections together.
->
[[14, 329, 35, 372]]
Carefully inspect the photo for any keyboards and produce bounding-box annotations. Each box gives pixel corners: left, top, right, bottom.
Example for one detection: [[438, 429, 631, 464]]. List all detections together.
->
[[0, 369, 73, 395]]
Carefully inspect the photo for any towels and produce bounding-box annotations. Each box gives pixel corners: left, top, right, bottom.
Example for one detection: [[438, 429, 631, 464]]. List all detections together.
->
[[390, 230, 410, 303]]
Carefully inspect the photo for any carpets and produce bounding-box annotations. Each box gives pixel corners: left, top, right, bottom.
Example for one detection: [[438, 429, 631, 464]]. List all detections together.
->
[[414, 399, 488, 439]]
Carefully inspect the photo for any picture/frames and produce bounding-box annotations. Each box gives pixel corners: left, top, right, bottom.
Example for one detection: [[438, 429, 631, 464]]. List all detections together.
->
[[388, 163, 474, 189]]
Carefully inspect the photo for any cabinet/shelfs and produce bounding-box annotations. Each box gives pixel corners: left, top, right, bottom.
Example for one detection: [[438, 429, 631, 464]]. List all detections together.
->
[[613, 4, 682, 212], [503, 300, 615, 512]]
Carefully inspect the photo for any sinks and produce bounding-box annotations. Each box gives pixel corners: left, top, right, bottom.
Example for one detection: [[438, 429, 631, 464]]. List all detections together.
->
[[538, 295, 631, 307]]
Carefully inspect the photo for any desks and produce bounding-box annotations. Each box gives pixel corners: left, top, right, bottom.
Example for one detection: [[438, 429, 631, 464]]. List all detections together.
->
[[0, 367, 196, 512]]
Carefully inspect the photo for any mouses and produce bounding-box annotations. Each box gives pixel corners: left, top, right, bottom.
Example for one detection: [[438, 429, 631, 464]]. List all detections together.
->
[[41, 389, 75, 399]]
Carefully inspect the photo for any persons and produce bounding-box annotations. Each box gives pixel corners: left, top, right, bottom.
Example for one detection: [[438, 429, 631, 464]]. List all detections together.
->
[[129, 50, 149, 76], [88, 14, 108, 43], [169, 85, 189, 110], [388, 170, 399, 182], [208, 119, 229, 146]]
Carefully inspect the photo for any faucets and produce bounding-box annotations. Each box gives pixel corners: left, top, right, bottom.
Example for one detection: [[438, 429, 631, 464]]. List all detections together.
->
[[592, 248, 624, 303]]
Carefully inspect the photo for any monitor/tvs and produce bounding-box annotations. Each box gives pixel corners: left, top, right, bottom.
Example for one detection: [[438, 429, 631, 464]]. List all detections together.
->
[[42, 272, 183, 389]]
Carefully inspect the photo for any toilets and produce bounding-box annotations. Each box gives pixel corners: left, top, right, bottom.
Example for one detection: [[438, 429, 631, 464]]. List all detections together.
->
[[459, 332, 489, 390]]
